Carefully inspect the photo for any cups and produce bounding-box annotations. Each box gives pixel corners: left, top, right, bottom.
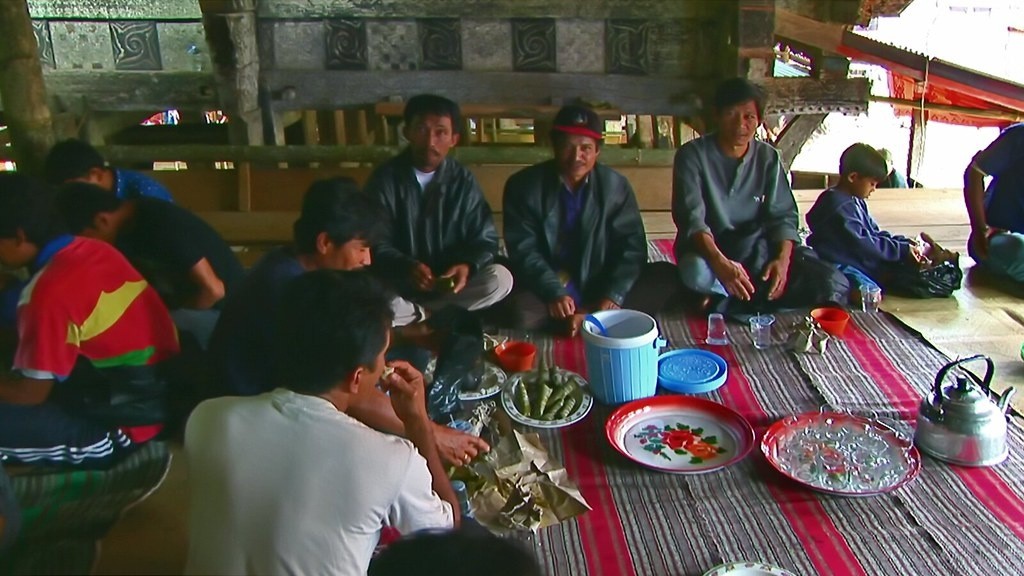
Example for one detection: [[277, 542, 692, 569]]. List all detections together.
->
[[776, 404, 913, 492], [811, 308, 849, 337], [447, 420, 474, 435], [706, 314, 727, 346], [860, 285, 881, 312], [750, 317, 771, 350]]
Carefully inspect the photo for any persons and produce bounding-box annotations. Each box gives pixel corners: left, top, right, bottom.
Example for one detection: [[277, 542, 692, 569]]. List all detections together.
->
[[495, 106, 682, 338], [963, 124, 1024, 282], [806, 143, 961, 300], [0, 94, 538, 576], [671, 78, 816, 321]]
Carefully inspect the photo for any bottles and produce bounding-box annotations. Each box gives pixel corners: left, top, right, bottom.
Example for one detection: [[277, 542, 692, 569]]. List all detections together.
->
[[448, 481, 474, 519], [188, 45, 202, 71]]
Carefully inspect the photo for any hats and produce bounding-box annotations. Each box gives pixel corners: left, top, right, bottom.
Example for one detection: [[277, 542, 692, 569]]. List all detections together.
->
[[552, 104, 604, 141]]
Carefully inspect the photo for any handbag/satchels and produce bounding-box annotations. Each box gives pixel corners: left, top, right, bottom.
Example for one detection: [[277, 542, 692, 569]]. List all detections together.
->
[[900, 244, 963, 299]]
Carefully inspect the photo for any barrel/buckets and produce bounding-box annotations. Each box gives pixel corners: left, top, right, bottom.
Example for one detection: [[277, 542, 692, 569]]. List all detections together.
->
[[582, 309, 667, 407]]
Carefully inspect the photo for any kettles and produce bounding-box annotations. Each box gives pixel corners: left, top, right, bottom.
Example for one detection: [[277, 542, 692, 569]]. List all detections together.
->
[[913, 354, 1017, 467]]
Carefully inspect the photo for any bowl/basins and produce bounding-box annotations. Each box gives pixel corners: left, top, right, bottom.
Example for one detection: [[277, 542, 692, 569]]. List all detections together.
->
[[495, 341, 537, 371]]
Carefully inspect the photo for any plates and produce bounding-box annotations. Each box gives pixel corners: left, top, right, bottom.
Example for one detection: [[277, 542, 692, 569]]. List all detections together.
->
[[501, 367, 593, 428], [423, 358, 506, 401], [702, 560, 796, 576]]
